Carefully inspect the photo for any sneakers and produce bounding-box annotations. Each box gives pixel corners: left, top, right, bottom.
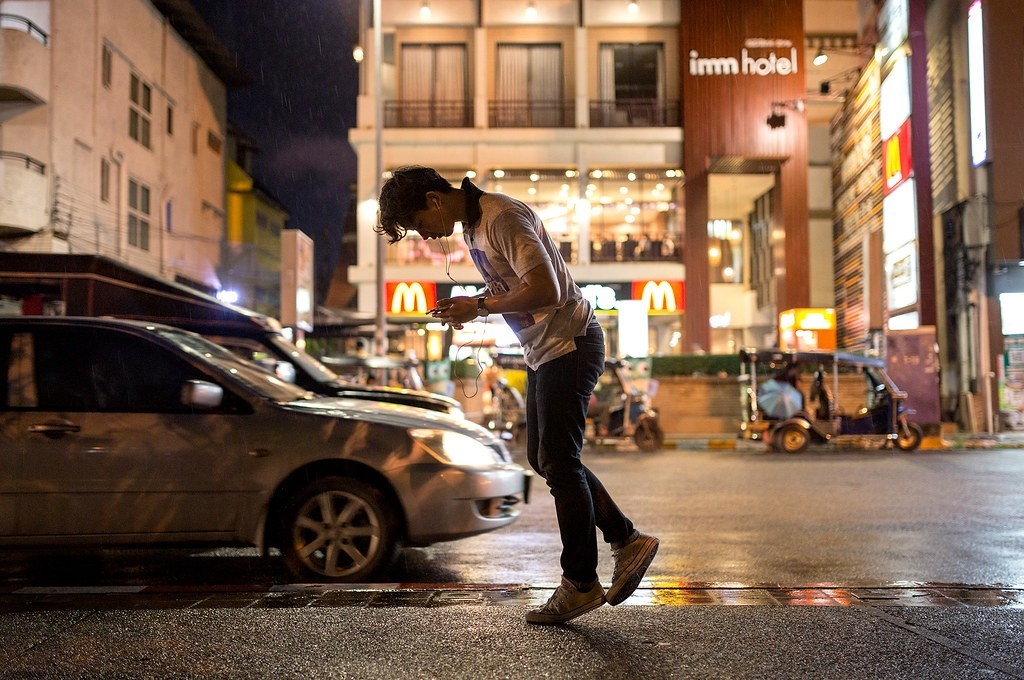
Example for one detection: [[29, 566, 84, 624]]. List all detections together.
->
[[525, 575, 606, 624], [606, 530, 660, 606]]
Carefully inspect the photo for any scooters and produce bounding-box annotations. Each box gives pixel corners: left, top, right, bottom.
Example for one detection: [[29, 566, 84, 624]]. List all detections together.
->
[[580, 355, 665, 451]]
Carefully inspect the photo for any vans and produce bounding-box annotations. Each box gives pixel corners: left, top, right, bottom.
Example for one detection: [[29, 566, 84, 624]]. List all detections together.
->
[[0, 248, 466, 417]]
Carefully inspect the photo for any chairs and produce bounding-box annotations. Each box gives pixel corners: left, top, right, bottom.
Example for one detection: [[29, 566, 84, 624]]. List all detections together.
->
[[651, 240, 663, 257], [559, 242, 572, 262], [601, 242, 618, 261], [622, 240, 636, 261]]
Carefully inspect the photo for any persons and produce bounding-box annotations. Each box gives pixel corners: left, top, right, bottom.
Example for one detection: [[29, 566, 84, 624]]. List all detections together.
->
[[373, 163, 661, 626], [774, 365, 800, 388]]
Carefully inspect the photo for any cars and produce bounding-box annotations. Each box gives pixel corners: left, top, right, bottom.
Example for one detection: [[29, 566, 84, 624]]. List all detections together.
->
[[0, 311, 535, 582]]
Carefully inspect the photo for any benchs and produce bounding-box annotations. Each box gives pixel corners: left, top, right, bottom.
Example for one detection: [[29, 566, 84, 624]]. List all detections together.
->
[[811, 372, 853, 434]]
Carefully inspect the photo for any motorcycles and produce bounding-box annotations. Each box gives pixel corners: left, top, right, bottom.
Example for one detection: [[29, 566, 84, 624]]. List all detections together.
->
[[739, 348, 923, 454]]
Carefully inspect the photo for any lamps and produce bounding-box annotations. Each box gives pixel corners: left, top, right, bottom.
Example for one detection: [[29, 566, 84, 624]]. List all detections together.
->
[[813, 42, 876, 66], [820, 66, 865, 95], [766, 98, 843, 130]]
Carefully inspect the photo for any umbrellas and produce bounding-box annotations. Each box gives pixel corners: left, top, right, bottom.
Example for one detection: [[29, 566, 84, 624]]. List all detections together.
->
[[758, 379, 803, 419]]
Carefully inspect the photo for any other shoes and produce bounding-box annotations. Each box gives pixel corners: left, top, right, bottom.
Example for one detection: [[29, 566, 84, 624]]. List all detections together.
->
[[599, 426, 609, 435]]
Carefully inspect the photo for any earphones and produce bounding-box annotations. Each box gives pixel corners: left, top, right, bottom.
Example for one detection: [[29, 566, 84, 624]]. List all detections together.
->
[[432, 198, 440, 209]]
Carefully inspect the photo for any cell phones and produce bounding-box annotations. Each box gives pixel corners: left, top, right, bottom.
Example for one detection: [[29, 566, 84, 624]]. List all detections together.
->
[[426, 308, 448, 315]]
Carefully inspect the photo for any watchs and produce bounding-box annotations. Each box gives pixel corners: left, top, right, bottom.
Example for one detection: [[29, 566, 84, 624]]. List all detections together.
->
[[477, 296, 489, 318]]
